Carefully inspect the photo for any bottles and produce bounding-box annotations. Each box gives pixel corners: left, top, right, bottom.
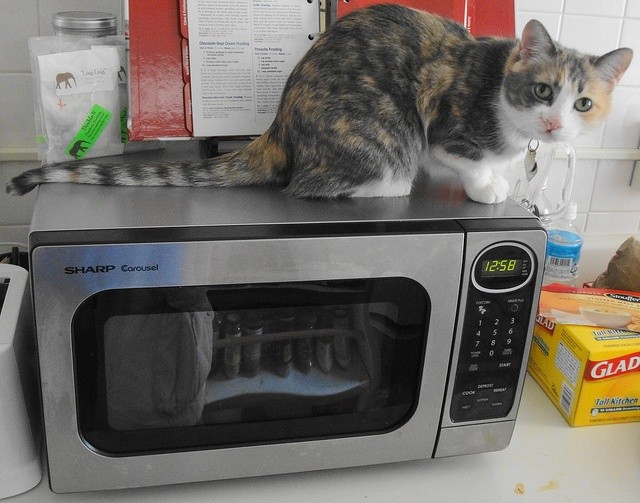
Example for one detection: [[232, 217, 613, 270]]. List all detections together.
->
[[223, 314, 244, 380], [244, 315, 264, 378], [316, 312, 336, 376], [209, 311, 223, 380], [270, 311, 296, 377], [296, 313, 317, 376], [542, 203, 585, 289], [334, 309, 352, 372]]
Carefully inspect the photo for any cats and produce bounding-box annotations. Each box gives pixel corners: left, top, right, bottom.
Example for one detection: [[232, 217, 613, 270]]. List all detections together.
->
[[3, 2, 635, 204]]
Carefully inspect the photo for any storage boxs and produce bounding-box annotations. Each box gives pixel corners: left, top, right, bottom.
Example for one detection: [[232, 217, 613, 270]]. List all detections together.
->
[[526, 285, 640, 426]]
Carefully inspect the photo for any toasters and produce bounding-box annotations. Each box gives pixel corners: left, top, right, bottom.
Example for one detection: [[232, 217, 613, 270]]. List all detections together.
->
[[0, 263, 44, 501]]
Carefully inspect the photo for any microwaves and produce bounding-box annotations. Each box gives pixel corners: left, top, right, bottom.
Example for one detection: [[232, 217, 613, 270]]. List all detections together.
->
[[26, 138, 548, 494]]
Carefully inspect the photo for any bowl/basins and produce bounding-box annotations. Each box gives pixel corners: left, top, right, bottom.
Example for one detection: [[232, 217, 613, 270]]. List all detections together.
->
[[580, 306, 634, 329]]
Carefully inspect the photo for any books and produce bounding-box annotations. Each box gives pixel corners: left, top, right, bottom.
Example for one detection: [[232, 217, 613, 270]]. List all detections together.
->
[[127, 0, 517, 140]]
